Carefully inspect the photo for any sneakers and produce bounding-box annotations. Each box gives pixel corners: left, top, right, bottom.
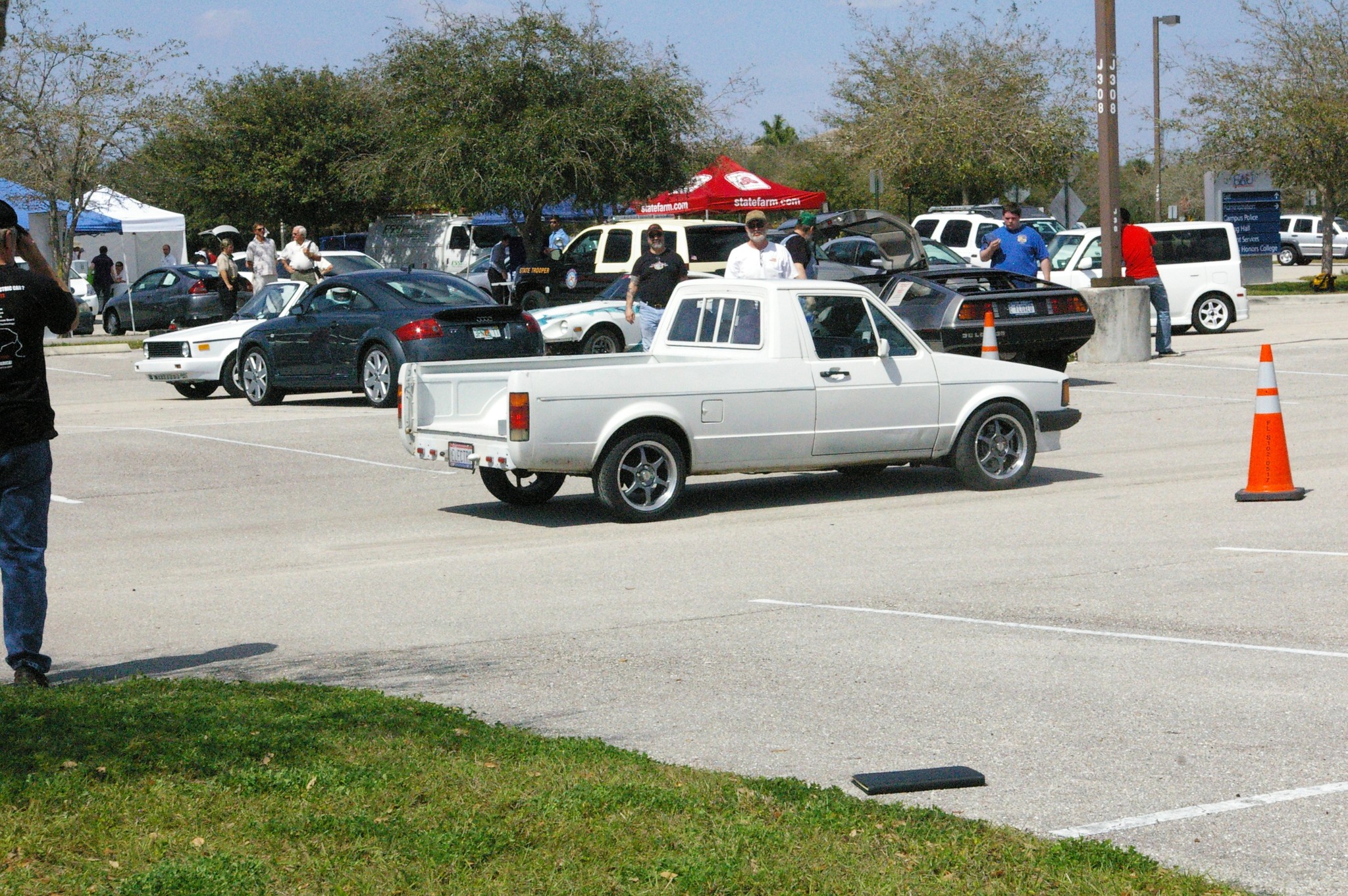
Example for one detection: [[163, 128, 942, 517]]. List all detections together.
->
[[1157, 350, 1182, 357]]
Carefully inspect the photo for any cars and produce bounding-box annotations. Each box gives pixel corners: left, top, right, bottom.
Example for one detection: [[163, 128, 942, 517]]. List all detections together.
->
[[102, 263, 254, 335], [271, 250, 407, 308], [237, 264, 549, 407], [140, 251, 282, 290], [14, 256, 99, 324], [530, 272, 725, 355], [762, 201, 1103, 294], [70, 287, 95, 335]]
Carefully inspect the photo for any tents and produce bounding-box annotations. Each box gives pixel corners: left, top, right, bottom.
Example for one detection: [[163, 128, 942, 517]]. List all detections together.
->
[[0, 177, 188, 335], [622, 154, 826, 219], [467, 191, 636, 281]]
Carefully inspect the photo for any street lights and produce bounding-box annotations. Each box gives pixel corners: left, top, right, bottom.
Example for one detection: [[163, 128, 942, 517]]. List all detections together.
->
[[1152, 15, 1180, 222]]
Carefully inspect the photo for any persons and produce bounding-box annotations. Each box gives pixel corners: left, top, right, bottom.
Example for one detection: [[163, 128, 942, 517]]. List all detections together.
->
[[624, 224, 689, 352], [216, 239, 238, 320], [89, 246, 121, 312], [112, 261, 127, 297], [980, 203, 1051, 290], [245, 223, 280, 294], [278, 225, 333, 310], [548, 215, 570, 251], [0, 200, 81, 686], [780, 211, 819, 334], [1119, 207, 1183, 356], [195, 247, 216, 265], [161, 245, 177, 266], [488, 233, 511, 304], [724, 210, 799, 279]]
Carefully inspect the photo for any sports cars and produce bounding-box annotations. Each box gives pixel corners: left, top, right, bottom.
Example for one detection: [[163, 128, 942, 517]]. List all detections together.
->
[[134, 279, 410, 400]]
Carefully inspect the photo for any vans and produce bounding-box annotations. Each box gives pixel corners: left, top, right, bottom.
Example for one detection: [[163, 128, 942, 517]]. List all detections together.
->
[[319, 232, 367, 254], [1036, 218, 1249, 339], [365, 213, 544, 301]]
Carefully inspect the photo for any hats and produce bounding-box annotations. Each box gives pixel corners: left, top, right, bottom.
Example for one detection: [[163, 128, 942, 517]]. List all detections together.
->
[[646, 224, 663, 236], [195, 250, 209, 265], [796, 210, 820, 232], [0, 199, 18, 229], [745, 210, 768, 225]]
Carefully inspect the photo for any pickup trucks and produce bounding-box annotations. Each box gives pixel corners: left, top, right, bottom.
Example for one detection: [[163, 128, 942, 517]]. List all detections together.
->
[[396, 278, 1080, 524]]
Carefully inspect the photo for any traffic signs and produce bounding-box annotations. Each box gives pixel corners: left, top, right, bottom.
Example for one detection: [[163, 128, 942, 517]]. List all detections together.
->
[[1224, 202, 1282, 212], [1223, 192, 1281, 206], [1223, 212, 1281, 255]]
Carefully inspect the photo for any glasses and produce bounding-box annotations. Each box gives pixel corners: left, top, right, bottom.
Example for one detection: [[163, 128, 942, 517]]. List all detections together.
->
[[550, 221, 557, 224], [115, 265, 121, 267], [228, 245, 235, 249], [748, 222, 764, 228], [291, 233, 299, 235], [254, 228, 265, 231], [649, 233, 662, 238]]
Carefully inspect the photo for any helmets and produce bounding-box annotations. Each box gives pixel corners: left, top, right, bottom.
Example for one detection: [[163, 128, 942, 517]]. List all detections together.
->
[[330, 287, 352, 306]]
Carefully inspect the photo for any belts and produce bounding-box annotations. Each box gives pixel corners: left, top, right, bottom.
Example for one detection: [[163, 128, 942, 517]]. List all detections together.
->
[[640, 298, 668, 310], [295, 268, 314, 275]]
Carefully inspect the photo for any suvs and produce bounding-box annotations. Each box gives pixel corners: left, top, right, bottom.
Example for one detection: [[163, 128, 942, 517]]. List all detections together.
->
[[1278, 214, 1348, 261], [549, 212, 749, 275]]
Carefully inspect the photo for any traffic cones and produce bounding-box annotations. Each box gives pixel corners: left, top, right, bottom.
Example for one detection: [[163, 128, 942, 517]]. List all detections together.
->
[[1233, 344, 1309, 503]]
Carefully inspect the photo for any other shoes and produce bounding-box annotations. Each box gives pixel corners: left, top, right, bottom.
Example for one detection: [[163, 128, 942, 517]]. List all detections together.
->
[[14, 658, 50, 688]]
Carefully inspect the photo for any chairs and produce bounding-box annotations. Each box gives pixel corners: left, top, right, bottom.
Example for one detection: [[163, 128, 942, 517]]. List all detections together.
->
[[676, 306, 719, 342], [731, 314, 760, 344]]
